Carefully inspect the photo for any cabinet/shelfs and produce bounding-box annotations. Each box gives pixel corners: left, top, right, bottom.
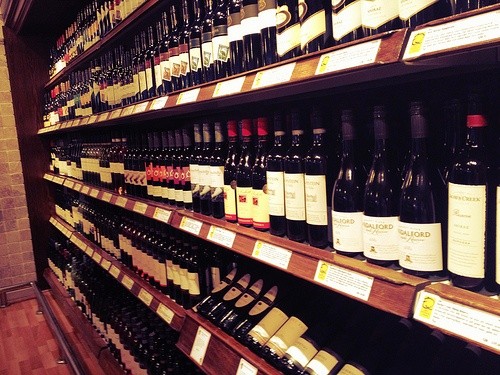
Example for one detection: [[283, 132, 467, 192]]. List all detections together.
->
[[37, 1, 500, 375]]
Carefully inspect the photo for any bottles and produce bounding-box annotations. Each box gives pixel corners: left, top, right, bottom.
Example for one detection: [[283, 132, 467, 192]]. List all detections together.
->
[[41, 0, 500, 375]]
